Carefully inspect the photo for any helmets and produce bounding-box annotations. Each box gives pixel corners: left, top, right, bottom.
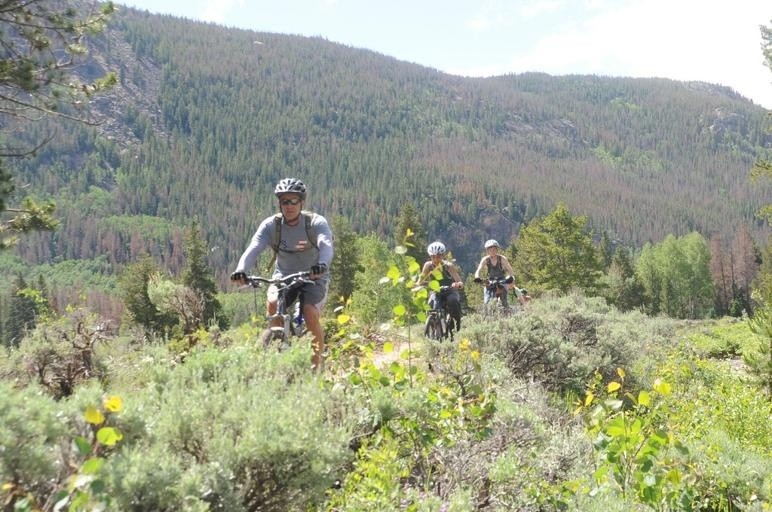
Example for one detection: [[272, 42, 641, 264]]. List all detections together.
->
[[274, 177, 306, 199], [427, 241, 446, 256], [484, 239, 499, 249]]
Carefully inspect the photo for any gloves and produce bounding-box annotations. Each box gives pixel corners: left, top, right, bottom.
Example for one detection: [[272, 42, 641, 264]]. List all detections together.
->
[[311, 263, 327, 274], [231, 270, 246, 281]]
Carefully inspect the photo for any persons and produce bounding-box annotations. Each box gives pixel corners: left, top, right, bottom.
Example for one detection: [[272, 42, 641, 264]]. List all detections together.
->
[[414, 241, 464, 347], [472, 238, 532, 314], [231, 178, 334, 376]]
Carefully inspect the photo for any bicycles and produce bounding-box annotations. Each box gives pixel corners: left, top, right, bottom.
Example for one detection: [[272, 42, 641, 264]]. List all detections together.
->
[[472, 275, 514, 320], [237, 268, 327, 354], [417, 281, 463, 343]]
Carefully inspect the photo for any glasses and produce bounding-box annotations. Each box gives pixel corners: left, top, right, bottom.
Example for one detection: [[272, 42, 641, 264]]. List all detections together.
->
[[278, 198, 301, 205]]
